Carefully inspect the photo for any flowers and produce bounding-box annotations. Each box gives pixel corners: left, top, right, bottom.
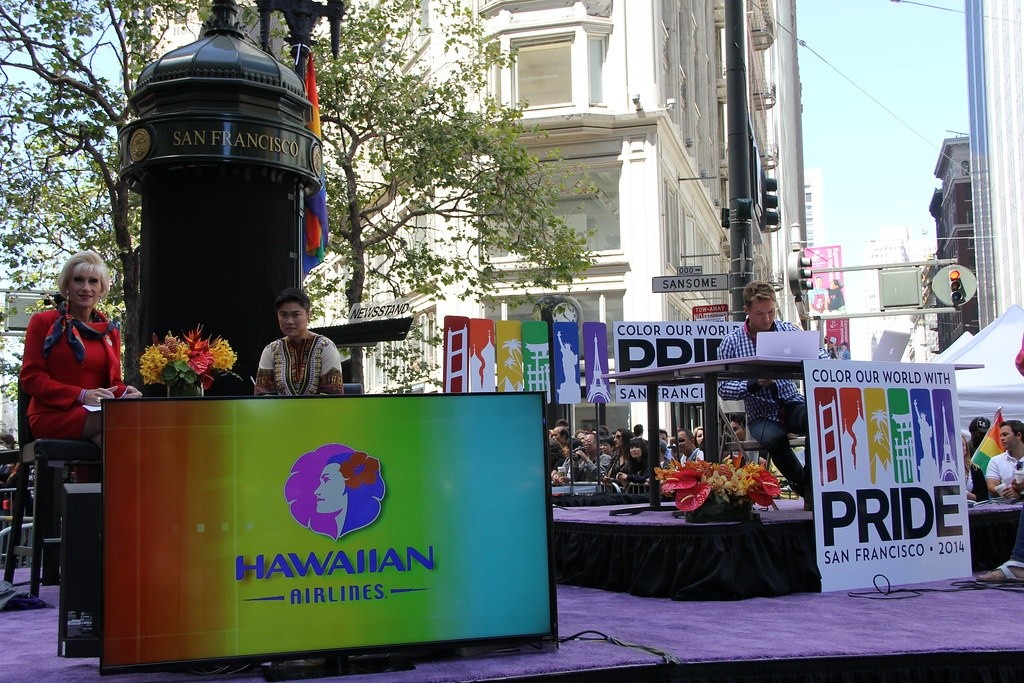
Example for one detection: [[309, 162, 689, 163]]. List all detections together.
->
[[654, 451, 782, 512], [139, 324, 237, 390]]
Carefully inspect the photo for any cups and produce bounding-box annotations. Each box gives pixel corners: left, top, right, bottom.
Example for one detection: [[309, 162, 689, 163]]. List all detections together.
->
[[1014, 470, 1024, 494], [557, 467, 567, 479], [994, 483, 1016, 504]]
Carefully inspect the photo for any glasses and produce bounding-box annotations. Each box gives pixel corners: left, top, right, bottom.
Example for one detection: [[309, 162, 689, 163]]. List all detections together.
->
[[678, 437, 686, 442], [613, 435, 622, 439]]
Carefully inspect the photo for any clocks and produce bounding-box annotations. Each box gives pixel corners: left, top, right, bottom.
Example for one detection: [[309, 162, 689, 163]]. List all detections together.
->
[[552, 303, 579, 323]]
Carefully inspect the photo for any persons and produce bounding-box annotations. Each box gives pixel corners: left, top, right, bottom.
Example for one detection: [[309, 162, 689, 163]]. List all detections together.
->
[[961, 417, 1024, 499], [975, 332, 1024, 586], [717, 281, 832, 511], [0, 432, 15, 487], [19, 252, 143, 448], [809, 276, 845, 313], [829, 336, 850, 360], [254, 287, 344, 397], [549, 414, 751, 493]]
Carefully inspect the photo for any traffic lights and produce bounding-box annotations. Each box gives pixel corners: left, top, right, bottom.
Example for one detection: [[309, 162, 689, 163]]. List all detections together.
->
[[949, 269, 964, 302], [760, 169, 779, 231], [800, 256, 814, 291]]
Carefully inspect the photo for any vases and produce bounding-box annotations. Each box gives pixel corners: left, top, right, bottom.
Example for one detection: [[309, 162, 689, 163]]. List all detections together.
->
[[683, 494, 752, 523], [166, 381, 204, 398]]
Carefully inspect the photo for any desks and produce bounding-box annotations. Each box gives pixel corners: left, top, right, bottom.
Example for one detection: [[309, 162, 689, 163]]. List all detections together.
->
[[602, 356, 984, 518]]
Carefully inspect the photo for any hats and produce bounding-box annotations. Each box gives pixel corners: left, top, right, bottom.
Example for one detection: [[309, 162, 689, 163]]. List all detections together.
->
[[969, 416, 991, 433]]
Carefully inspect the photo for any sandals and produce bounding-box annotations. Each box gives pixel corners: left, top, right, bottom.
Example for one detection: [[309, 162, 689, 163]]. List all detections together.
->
[[978, 562, 1024, 582]]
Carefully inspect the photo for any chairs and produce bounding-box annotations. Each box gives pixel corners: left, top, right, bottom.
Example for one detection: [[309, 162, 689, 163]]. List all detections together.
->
[[4, 374, 102, 597], [718, 400, 806, 512]]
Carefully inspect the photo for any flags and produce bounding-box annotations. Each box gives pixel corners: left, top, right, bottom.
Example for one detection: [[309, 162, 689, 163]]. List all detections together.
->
[[971, 413, 1004, 501], [301, 52, 329, 274]]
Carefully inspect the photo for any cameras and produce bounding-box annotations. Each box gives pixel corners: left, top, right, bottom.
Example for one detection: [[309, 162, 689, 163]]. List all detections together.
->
[[573, 446, 585, 461]]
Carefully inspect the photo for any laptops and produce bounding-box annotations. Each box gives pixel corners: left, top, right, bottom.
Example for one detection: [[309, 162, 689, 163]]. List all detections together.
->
[[872, 329, 911, 362], [756, 331, 819, 359]]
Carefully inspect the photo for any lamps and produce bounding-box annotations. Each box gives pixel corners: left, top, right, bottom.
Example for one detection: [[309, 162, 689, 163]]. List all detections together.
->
[[700, 170, 708, 177], [665, 98, 676, 110], [632, 93, 640, 105], [595, 188, 619, 215], [714, 199, 721, 206], [686, 138, 693, 147]]
